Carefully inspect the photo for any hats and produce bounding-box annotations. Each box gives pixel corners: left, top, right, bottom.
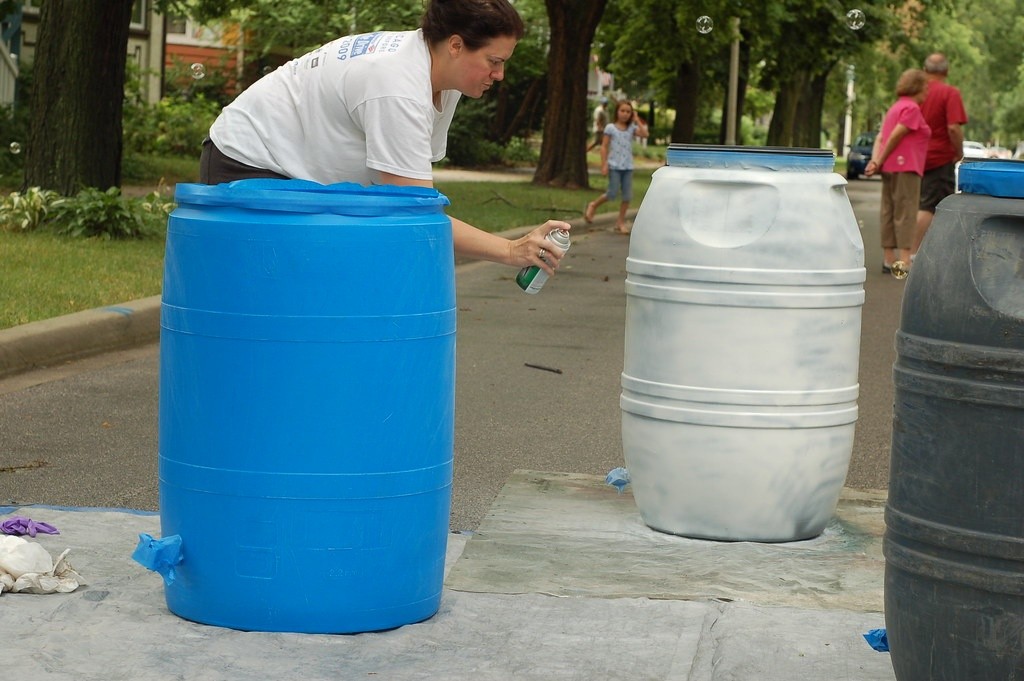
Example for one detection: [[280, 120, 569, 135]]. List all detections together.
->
[[601, 98, 608, 103]]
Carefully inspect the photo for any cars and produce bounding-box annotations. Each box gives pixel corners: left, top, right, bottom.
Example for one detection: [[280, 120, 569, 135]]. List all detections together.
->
[[847, 132, 882, 177], [962, 140, 1002, 161]]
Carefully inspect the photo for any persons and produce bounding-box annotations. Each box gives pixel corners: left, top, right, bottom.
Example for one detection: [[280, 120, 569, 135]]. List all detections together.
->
[[585, 97, 609, 152], [583, 100, 648, 235], [864, 67, 932, 277], [201, 0, 570, 276], [908, 53, 967, 263]]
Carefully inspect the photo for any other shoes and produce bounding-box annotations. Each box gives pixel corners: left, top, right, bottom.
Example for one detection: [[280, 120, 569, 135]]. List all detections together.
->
[[883, 263, 891, 273], [585, 201, 596, 224], [615, 224, 630, 233]]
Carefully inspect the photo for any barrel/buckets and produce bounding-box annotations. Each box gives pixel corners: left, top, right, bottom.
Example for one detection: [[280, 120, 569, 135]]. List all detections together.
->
[[618, 144, 867, 542], [157, 179, 458, 634], [884, 156, 1024, 681]]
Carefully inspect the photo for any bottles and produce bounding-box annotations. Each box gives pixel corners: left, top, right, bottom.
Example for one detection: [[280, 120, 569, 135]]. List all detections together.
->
[[514, 228, 571, 295]]
